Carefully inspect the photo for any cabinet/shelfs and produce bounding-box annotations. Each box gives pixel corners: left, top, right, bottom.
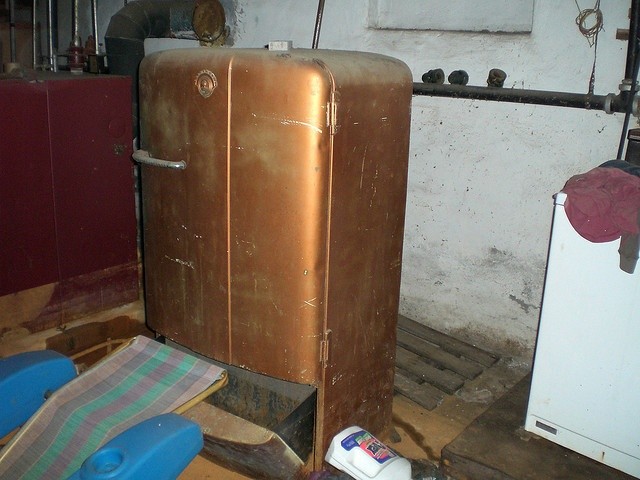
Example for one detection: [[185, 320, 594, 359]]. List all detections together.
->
[[1, 75, 139, 345]]
